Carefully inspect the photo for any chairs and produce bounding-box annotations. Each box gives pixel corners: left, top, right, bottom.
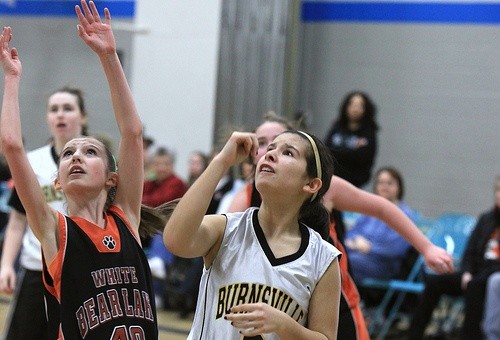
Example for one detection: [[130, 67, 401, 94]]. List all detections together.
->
[[361, 213, 478, 340]]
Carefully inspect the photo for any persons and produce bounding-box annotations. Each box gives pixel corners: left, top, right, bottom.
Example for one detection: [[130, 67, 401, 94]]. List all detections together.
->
[[398, 172, 500, 340], [325, 91, 380, 188], [227, 111, 455, 339], [0, 87, 89, 340], [0, 0, 160, 340], [163, 129, 343, 340], [481, 272, 500, 340], [345, 165, 419, 285], [0, 138, 25, 272], [141, 136, 253, 319]]
